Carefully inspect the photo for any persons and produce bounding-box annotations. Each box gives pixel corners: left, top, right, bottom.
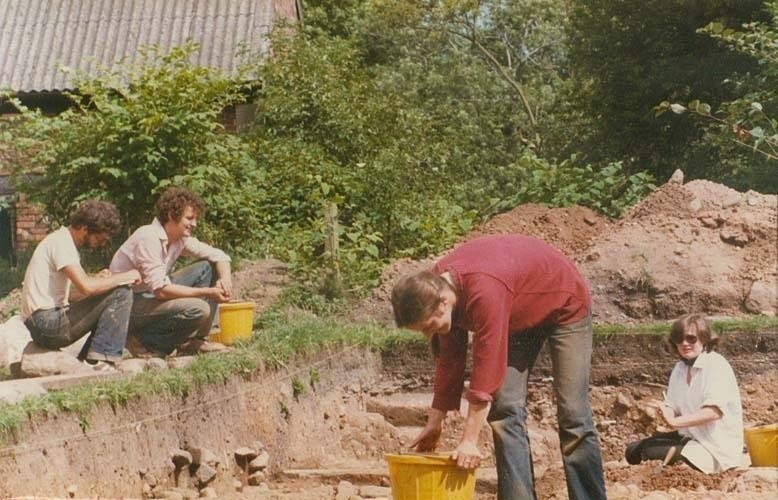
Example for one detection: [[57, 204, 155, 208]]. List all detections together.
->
[[624, 314, 744, 473], [391, 231, 606, 500], [19, 198, 142, 363], [112, 190, 234, 360]]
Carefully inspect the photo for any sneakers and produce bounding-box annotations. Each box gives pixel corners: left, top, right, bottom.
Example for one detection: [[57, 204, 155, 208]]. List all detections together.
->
[[123, 336, 226, 358], [84, 360, 122, 373]]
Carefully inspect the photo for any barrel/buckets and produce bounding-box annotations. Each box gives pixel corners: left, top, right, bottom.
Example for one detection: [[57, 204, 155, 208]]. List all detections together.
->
[[210, 301, 256, 344]]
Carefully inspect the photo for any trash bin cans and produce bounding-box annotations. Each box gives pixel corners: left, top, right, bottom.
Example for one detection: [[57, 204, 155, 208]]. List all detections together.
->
[[219, 301, 256, 345]]
